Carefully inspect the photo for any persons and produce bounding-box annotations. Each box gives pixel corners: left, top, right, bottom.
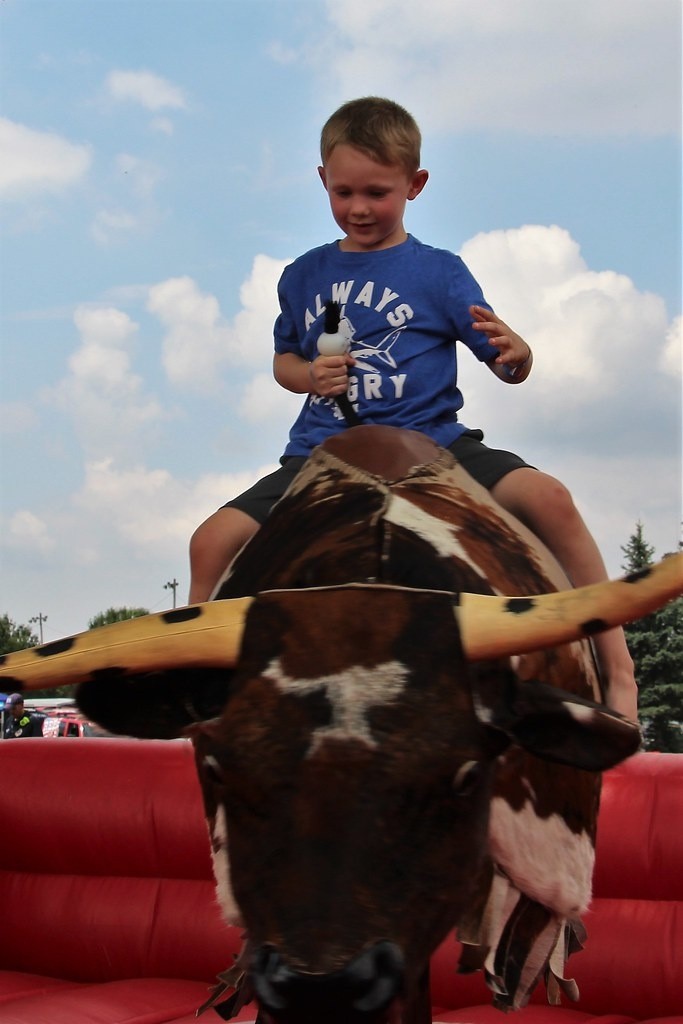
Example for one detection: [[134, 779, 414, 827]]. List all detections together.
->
[[4, 693, 43, 739], [188, 98, 638, 722]]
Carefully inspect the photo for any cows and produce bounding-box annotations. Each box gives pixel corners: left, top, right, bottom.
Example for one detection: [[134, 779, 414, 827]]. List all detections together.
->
[[0, 419, 683, 1023]]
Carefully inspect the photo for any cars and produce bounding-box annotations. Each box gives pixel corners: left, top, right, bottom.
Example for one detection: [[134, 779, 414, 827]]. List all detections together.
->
[[35, 706, 91, 738]]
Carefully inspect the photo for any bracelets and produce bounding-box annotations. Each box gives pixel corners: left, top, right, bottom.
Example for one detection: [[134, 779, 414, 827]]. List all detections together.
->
[[520, 342, 530, 366]]
